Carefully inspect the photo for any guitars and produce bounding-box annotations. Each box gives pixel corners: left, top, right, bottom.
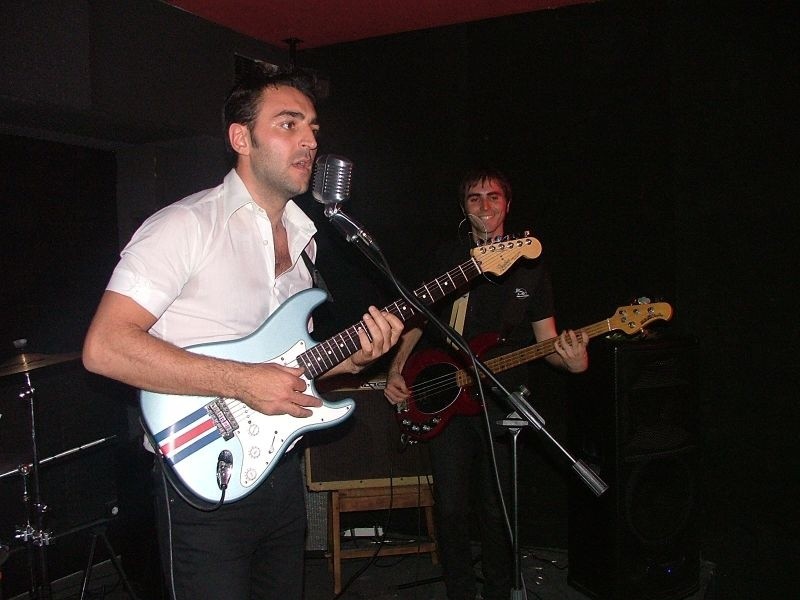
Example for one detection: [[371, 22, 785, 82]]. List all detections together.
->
[[393, 295, 672, 442], [135, 230, 544, 512]]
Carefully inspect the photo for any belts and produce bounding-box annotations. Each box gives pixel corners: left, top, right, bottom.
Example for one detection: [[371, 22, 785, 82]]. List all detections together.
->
[[277, 446, 296, 465]]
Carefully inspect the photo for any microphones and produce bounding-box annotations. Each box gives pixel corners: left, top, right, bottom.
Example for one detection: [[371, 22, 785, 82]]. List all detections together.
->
[[312, 154, 354, 217]]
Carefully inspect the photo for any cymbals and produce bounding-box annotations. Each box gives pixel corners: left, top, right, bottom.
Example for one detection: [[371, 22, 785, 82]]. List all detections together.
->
[[0, 351, 82, 376]]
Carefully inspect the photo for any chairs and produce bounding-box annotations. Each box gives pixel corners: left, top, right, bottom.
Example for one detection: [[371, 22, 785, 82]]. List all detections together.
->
[[328, 483, 440, 593]]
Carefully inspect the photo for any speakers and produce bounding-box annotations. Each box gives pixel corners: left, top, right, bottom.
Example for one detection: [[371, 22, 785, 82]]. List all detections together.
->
[[567, 333, 703, 600]]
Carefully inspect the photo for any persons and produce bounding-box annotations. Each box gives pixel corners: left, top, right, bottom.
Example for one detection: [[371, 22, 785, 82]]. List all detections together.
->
[[83, 68, 406, 600], [385, 165, 588, 600]]
